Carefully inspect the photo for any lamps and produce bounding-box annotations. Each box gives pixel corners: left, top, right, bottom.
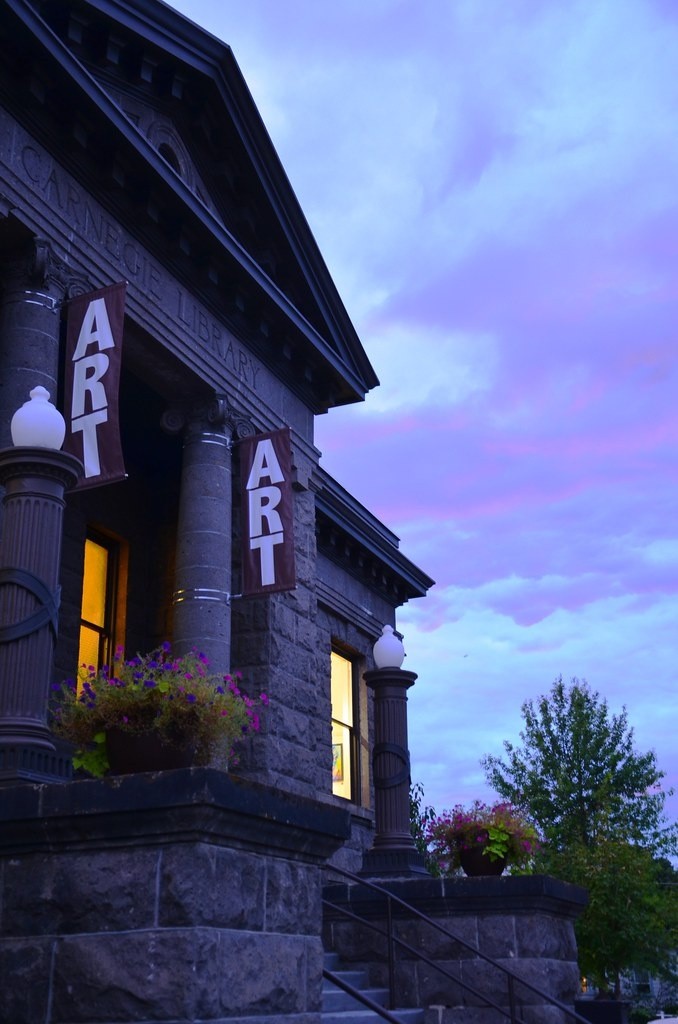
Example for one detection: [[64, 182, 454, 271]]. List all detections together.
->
[[450, 831, 511, 876], [104, 716, 213, 775]]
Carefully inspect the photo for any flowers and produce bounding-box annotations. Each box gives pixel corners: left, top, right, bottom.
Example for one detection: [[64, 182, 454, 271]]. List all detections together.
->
[[47, 641, 269, 766], [417, 798, 546, 874]]
